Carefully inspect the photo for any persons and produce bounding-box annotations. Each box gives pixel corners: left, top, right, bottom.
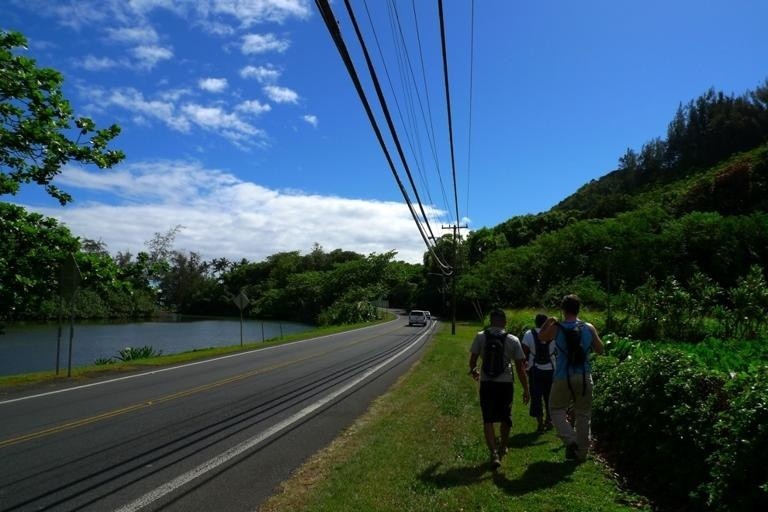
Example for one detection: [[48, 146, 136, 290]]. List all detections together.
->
[[536, 293, 604, 466], [464, 309, 530, 469], [519, 313, 558, 436]]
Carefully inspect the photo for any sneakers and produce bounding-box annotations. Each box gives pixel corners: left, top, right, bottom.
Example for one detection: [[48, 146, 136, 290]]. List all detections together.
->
[[537, 419, 553, 433], [566, 442, 586, 461], [491, 446, 508, 467]]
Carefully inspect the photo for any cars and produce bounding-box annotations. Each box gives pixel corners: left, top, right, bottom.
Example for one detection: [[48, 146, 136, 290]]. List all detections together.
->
[[408, 310, 431, 326]]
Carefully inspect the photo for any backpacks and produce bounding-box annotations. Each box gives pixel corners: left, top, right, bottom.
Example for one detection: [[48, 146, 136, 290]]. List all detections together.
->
[[531, 328, 555, 371], [557, 320, 585, 367], [482, 330, 508, 377]]
